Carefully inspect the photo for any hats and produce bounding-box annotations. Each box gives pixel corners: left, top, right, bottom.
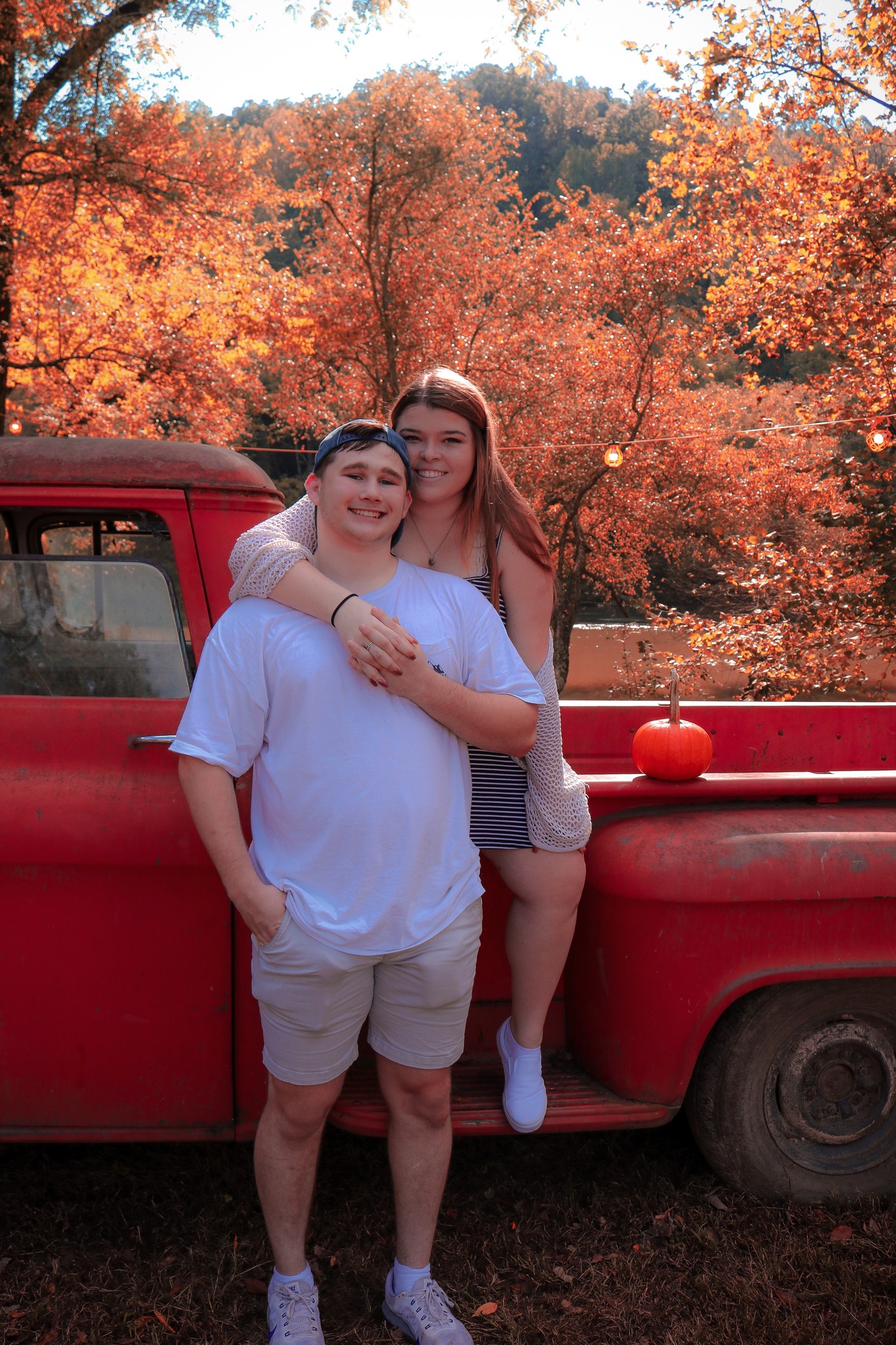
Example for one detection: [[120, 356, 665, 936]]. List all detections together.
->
[[312, 419, 411, 548]]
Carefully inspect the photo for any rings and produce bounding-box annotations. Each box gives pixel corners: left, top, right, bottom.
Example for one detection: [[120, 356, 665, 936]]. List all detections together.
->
[[367, 642, 373, 650]]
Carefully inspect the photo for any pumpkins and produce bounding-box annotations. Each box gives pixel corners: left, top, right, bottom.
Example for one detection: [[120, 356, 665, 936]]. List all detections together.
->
[[632, 668, 712, 781]]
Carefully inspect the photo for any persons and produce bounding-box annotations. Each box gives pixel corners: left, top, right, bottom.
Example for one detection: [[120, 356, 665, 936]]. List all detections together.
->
[[169, 421, 549, 1345], [230, 366, 591, 1133]]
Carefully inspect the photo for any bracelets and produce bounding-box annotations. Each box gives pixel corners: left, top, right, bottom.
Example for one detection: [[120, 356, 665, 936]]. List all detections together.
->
[[331, 593, 358, 627]]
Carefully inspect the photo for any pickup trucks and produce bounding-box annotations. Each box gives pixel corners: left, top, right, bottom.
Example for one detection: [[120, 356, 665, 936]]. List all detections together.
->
[[1, 432, 896, 1217]]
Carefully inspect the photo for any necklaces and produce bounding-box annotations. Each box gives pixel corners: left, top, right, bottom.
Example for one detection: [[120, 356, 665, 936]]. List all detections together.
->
[[409, 502, 461, 565]]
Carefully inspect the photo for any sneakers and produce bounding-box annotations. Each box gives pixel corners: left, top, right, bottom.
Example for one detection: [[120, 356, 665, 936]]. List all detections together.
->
[[381, 1268, 473, 1345], [267, 1276, 325, 1345], [496, 1016, 548, 1132]]
